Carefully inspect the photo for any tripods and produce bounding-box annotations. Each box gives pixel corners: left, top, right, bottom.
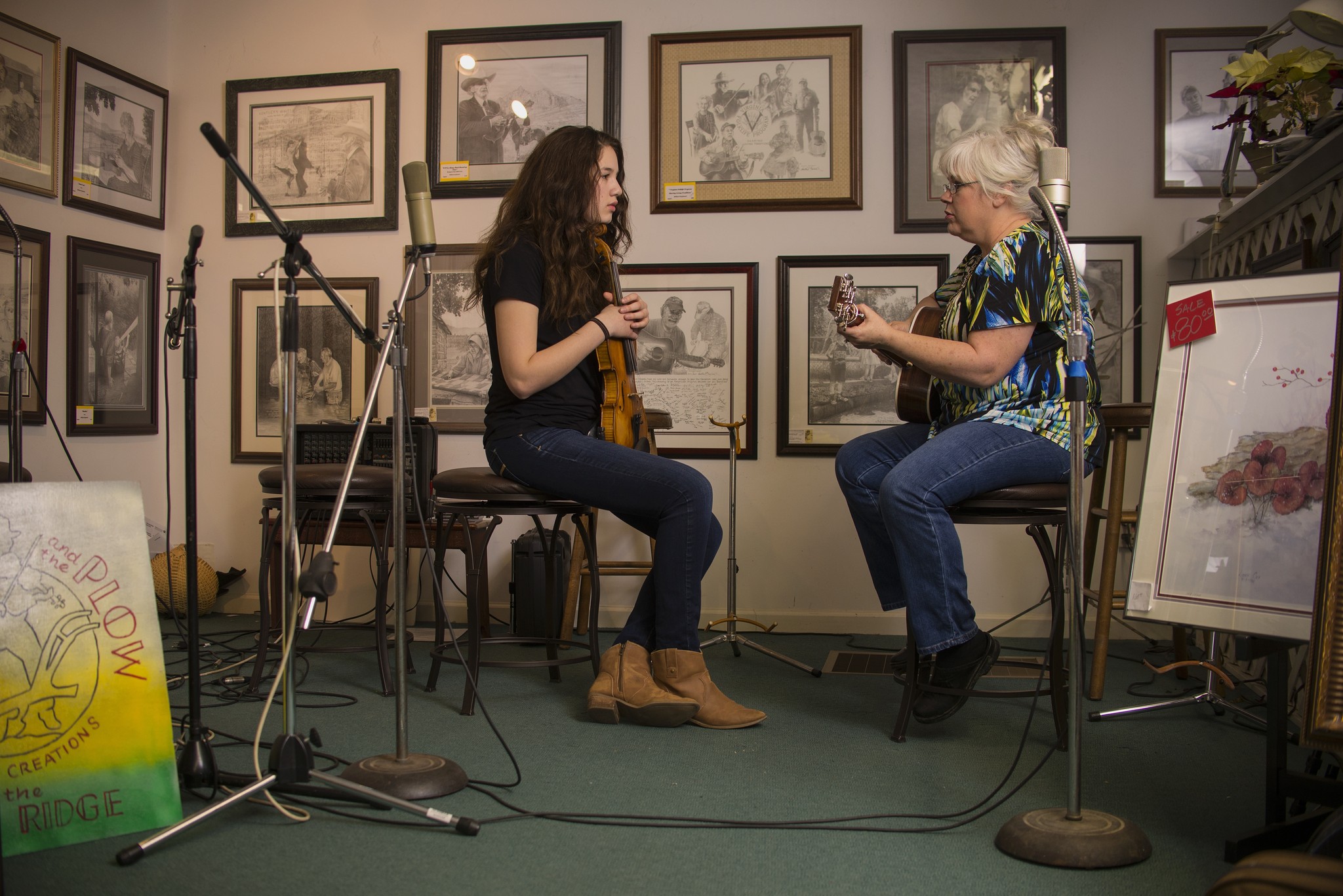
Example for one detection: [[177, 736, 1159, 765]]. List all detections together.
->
[[1088, 630, 1302, 744], [113, 120, 482, 865], [701, 449, 823, 679]]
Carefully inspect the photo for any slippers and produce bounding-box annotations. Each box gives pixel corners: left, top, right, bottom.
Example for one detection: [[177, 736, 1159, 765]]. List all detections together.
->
[[911, 631, 1000, 724], [887, 635, 917, 667]]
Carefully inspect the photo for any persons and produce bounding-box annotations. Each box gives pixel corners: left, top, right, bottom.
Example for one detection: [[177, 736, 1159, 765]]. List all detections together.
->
[[463, 124, 766, 729], [835, 109, 1105, 721]]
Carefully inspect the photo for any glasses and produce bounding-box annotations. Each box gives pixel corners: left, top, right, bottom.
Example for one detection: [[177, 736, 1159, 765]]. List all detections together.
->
[[942, 180, 979, 195]]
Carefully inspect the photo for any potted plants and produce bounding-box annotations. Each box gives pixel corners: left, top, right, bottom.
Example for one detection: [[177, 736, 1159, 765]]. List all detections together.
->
[[1202, 46, 1342, 182]]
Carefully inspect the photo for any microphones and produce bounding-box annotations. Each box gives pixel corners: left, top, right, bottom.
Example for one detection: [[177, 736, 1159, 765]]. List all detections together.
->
[[1037, 146, 1071, 222], [403, 160, 437, 287]]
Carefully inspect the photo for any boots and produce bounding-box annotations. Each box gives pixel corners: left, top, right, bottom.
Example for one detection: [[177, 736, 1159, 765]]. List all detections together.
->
[[586, 640, 700, 727], [649, 648, 768, 728]]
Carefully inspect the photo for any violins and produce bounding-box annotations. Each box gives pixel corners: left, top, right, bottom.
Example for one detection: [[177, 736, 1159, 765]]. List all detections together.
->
[[589, 224, 651, 456]]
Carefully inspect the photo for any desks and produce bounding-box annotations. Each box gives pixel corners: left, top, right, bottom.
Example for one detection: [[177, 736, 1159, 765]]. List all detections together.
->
[[258, 517, 503, 638]]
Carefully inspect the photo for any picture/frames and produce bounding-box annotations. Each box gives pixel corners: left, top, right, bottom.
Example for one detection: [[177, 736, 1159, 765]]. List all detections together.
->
[[650, 25, 864, 214], [1122, 266, 1341, 644], [0, 11, 62, 198], [1066, 235, 1143, 440], [65, 235, 161, 435], [777, 253, 951, 455], [61, 43, 169, 231], [225, 67, 399, 237], [616, 261, 758, 458], [425, 19, 623, 200], [1153, 24, 1270, 201], [0, 219, 50, 424], [892, 25, 1070, 233], [231, 276, 382, 460], [403, 243, 490, 433]]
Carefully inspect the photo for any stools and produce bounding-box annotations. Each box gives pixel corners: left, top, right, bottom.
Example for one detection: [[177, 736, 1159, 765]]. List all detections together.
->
[[889, 482, 1069, 751], [243, 463, 412, 697], [1084, 403, 1189, 701], [551, 407, 672, 648], [421, 465, 601, 717]]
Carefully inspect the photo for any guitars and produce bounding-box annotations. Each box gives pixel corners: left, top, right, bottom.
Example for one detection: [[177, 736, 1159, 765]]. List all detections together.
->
[[829, 273, 946, 424]]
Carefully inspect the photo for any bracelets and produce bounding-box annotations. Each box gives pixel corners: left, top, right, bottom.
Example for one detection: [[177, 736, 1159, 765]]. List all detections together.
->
[[589, 317, 610, 340]]
